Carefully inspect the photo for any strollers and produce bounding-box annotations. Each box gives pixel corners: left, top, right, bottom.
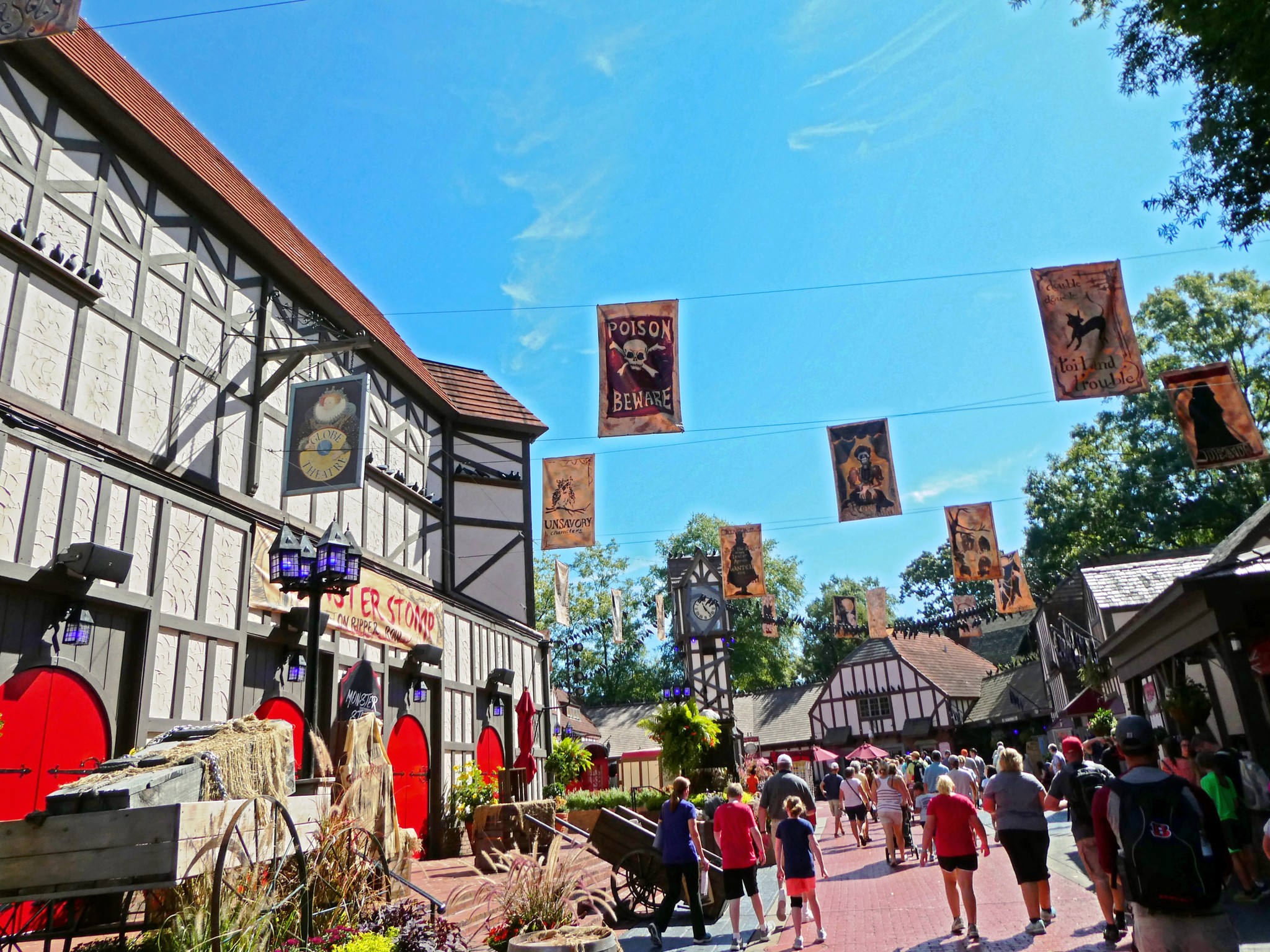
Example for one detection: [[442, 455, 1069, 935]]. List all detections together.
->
[[884, 803, 919, 863]]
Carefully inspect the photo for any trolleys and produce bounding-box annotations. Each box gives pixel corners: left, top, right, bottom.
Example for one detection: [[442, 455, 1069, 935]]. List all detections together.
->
[[0, 774, 394, 952], [523, 804, 729, 923]]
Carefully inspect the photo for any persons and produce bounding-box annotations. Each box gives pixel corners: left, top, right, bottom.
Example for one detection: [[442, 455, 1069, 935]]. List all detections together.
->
[[745, 765, 775, 799], [647, 776, 712, 948], [820, 716, 1270, 952], [759, 754, 816, 922], [714, 783, 775, 951], [775, 795, 829, 950]]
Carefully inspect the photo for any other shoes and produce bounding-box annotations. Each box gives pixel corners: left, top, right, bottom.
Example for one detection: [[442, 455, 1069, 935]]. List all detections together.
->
[[1242, 880, 1268, 897], [834, 815, 935, 867]]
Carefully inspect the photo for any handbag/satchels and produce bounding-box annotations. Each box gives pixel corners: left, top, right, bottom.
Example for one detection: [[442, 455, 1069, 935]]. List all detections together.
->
[[652, 804, 665, 850], [863, 802, 868, 815]]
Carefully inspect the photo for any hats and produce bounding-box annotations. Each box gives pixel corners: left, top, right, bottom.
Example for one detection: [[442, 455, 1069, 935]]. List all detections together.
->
[[777, 754, 792, 763], [1115, 715, 1156, 750], [1062, 737, 1081, 753], [909, 749, 968, 765], [1111, 727, 1116, 735]]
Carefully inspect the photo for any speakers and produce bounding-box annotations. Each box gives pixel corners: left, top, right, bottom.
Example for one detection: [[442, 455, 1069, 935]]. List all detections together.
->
[[64, 541, 134, 584], [287, 607, 329, 636], [491, 668, 515, 685], [412, 644, 443, 666]]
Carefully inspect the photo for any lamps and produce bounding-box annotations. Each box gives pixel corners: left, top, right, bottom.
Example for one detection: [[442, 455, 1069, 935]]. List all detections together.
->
[[52, 599, 95, 645], [488, 668, 515, 693], [408, 644, 443, 674], [488, 694, 504, 716], [279, 649, 307, 686], [58, 541, 134, 584], [285, 607, 329, 636], [404, 674, 428, 706], [553, 720, 573, 739]]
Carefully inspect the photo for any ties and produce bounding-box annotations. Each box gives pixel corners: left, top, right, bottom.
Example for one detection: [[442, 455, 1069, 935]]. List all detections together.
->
[[831, 762, 839, 769]]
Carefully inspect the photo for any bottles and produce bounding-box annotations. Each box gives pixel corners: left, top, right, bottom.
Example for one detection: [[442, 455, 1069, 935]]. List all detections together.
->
[[1199, 829, 1212, 857], [1116, 849, 1132, 901]]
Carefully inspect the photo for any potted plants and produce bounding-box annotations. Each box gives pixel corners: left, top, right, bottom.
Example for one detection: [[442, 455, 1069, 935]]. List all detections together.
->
[[544, 782, 568, 833], [450, 760, 505, 855]]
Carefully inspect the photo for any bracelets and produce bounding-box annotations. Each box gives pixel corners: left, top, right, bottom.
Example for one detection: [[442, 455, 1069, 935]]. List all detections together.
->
[[920, 848, 928, 852], [760, 831, 767, 834]]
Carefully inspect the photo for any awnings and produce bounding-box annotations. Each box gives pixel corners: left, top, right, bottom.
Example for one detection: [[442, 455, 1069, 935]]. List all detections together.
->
[[823, 726, 852, 745], [901, 716, 933, 738]]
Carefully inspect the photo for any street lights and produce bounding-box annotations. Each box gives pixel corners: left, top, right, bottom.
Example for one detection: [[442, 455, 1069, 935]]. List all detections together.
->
[[267, 512, 364, 780]]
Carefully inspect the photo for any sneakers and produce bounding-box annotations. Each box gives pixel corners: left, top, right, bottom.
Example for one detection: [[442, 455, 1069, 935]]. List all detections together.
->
[[802, 909, 815, 923], [952, 917, 979, 938], [793, 935, 804, 948], [1026, 908, 1056, 934], [648, 922, 663, 947], [818, 928, 827, 941], [759, 920, 775, 940], [1104, 920, 1129, 940], [776, 896, 787, 922], [694, 932, 712, 943], [730, 936, 743, 950]]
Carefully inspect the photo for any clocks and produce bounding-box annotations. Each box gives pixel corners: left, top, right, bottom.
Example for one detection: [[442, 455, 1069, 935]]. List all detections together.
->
[[693, 597, 716, 620]]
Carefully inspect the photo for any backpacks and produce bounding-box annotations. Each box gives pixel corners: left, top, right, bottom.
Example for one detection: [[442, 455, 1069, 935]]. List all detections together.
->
[[1216, 746, 1270, 810], [912, 761, 925, 784], [1069, 763, 1114, 826], [1108, 773, 1223, 916]]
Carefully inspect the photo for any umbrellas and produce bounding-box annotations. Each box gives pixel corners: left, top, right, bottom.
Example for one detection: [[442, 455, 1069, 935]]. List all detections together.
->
[[845, 741, 889, 762], [514, 686, 536, 801], [810, 744, 839, 778]]
